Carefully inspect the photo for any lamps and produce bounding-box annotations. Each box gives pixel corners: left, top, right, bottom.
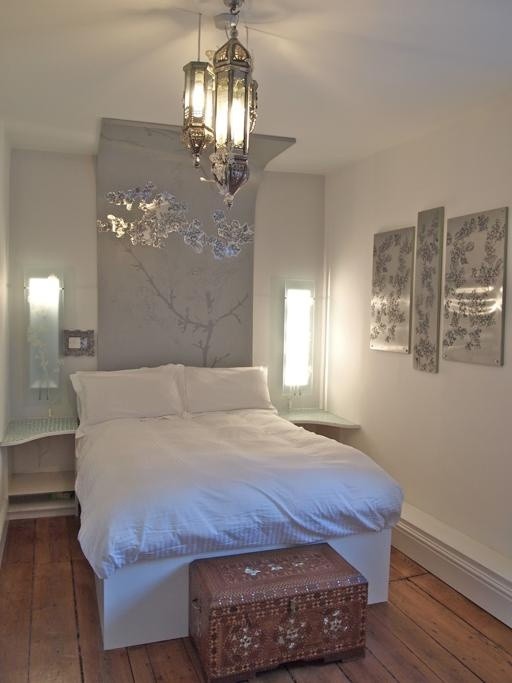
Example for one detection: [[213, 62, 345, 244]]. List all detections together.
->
[[180, 0, 260, 212]]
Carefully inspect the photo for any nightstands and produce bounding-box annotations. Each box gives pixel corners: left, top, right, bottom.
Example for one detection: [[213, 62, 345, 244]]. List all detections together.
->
[[278, 410, 360, 442], [1, 417, 80, 520]]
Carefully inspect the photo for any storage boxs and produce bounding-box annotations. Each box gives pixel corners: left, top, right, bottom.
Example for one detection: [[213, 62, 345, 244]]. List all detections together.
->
[[188, 541, 369, 682]]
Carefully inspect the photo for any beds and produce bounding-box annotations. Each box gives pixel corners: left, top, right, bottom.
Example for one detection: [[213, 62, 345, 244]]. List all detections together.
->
[[70, 364, 404, 650]]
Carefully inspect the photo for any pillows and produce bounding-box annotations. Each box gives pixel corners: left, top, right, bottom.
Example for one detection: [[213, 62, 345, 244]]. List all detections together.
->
[[69, 363, 280, 439]]
